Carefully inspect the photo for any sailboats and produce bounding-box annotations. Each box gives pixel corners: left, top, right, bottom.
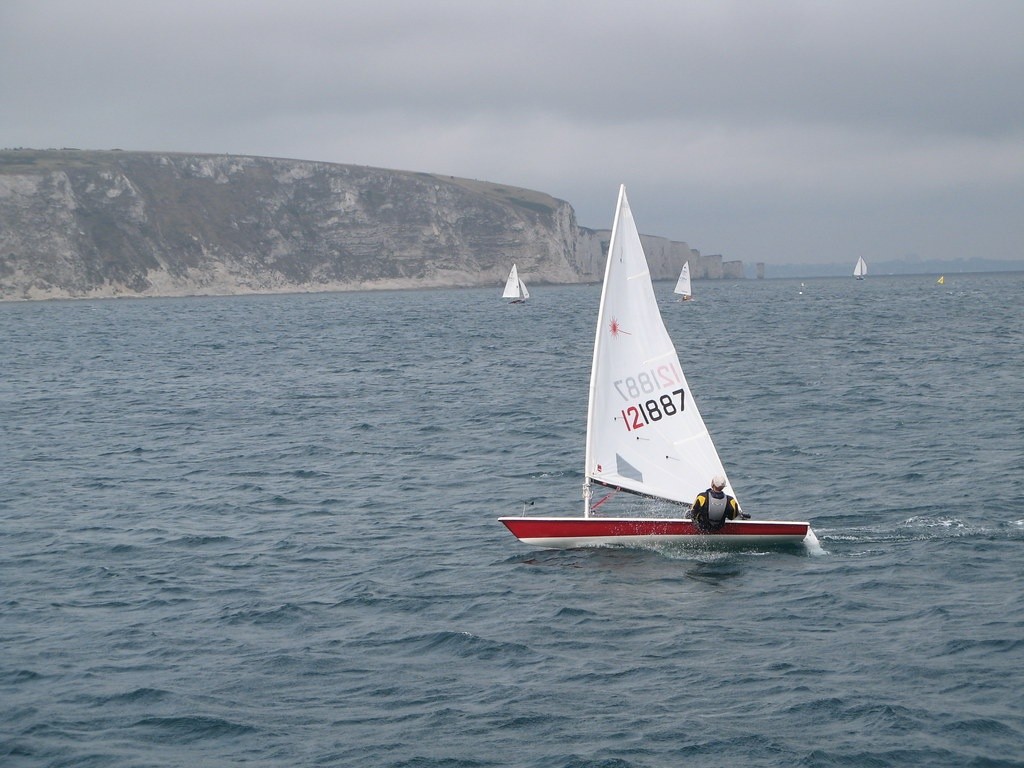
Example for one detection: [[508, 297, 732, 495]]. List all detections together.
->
[[673, 261, 692, 301], [500, 182, 811, 549], [853, 256, 868, 280], [501, 263, 529, 304]]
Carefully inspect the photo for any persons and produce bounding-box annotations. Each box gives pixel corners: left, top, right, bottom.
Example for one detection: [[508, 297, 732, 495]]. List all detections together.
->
[[690, 475, 739, 532]]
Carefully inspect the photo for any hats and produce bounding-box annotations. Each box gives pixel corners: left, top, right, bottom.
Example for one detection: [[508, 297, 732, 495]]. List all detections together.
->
[[713, 475, 726, 487]]
